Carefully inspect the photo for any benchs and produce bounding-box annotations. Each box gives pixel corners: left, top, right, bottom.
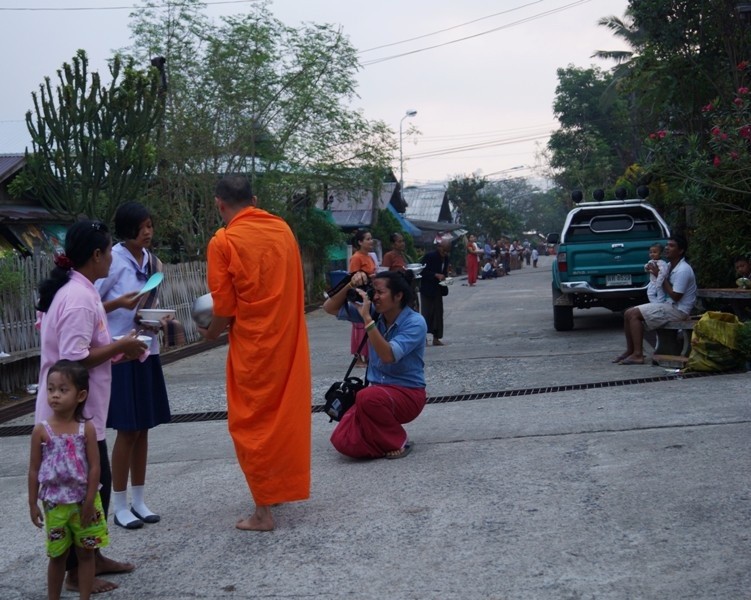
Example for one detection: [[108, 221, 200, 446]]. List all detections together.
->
[[649, 319, 704, 366]]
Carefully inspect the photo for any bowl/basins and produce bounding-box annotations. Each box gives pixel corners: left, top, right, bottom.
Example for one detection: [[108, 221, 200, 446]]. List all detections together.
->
[[138, 309, 177, 321], [191, 293, 214, 329], [111, 335, 152, 351]]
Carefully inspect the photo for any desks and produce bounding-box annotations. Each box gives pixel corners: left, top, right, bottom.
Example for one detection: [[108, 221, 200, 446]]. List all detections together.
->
[[696, 286, 751, 301]]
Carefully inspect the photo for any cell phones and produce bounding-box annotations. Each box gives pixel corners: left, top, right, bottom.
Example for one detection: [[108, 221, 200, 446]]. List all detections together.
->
[[139, 319, 161, 328]]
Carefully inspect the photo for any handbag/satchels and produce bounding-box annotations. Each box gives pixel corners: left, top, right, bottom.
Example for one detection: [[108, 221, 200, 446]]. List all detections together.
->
[[442, 286, 448, 296], [324, 377, 366, 422]]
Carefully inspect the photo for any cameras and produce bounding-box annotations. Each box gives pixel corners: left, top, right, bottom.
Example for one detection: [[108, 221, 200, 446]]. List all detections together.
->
[[346, 276, 375, 304]]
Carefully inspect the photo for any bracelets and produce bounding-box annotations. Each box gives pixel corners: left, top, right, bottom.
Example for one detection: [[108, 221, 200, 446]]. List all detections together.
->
[[364, 319, 376, 331], [348, 283, 354, 289]]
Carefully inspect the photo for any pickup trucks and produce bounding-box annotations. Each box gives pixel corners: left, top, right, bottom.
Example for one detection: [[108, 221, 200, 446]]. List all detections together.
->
[[547, 185, 672, 331]]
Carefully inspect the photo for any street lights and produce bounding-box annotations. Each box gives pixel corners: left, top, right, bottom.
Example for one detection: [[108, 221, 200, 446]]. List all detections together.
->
[[400, 108, 418, 199]]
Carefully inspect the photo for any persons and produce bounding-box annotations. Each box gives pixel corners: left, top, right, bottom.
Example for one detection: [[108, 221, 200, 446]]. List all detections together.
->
[[612, 235, 697, 365], [196, 174, 312, 530], [36, 220, 134, 593], [466, 234, 479, 287], [482, 238, 531, 279], [532, 246, 538, 268], [381, 233, 406, 272], [323, 269, 427, 460], [94, 201, 171, 527], [348, 230, 376, 367], [28, 359, 110, 600], [421, 231, 448, 346], [732, 254, 751, 290]]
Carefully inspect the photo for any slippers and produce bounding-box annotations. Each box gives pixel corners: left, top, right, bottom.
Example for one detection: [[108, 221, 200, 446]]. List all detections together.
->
[[612, 354, 625, 362], [388, 440, 414, 458], [619, 359, 645, 365]]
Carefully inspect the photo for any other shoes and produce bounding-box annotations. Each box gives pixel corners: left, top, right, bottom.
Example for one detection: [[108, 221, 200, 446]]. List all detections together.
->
[[130, 507, 160, 524], [433, 340, 442, 345], [114, 514, 144, 529]]
